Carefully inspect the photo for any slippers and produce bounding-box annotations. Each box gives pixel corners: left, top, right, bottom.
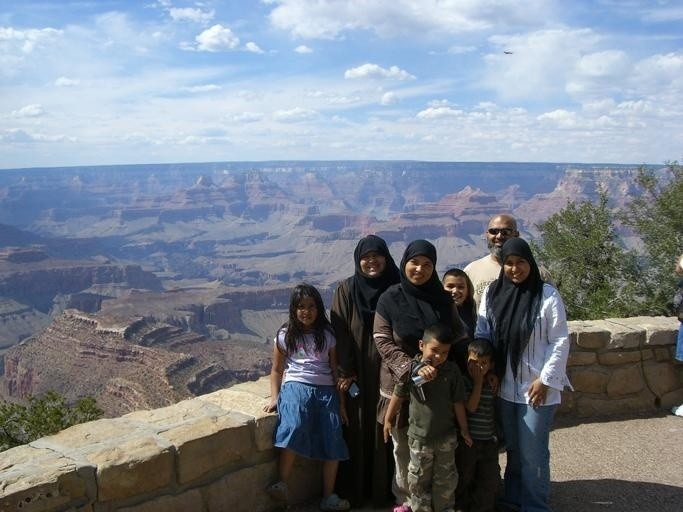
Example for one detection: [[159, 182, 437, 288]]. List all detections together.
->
[[319, 495, 349, 511], [267, 481, 288, 501]]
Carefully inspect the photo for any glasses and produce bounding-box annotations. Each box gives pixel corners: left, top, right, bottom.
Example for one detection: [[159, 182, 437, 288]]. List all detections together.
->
[[488, 229, 512, 236]]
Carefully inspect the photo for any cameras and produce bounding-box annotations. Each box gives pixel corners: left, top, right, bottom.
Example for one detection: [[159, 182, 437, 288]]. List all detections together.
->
[[346, 380, 360, 398], [410, 375, 430, 402]]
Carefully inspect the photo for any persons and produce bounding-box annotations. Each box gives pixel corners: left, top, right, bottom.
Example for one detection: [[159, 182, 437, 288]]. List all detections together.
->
[[263, 282, 351, 512], [464, 213, 560, 318], [331, 234, 400, 511], [372, 240, 464, 512], [441, 269, 478, 333], [382, 323, 472, 512], [474, 236, 574, 512], [455, 338, 501, 512], [669, 252, 683, 418]]
